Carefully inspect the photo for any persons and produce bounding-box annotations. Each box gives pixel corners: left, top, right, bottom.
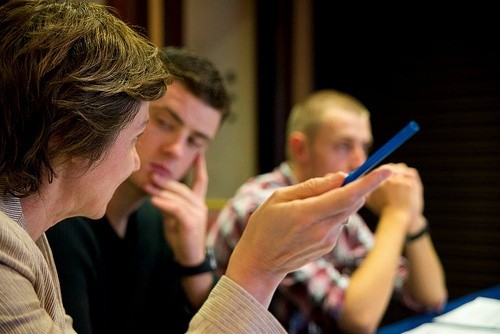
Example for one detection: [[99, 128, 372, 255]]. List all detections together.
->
[[45, 47, 231, 334], [205, 90, 447, 334], [0, 0, 393, 334]]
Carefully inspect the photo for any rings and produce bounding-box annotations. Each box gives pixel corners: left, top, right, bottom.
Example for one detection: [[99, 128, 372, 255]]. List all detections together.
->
[[344, 217, 350, 224]]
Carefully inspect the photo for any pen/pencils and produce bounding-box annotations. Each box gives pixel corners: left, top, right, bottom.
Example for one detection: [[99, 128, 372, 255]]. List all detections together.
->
[[341, 122, 419, 186]]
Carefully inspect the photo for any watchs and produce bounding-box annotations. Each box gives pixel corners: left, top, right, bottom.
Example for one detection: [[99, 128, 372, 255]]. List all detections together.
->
[[177, 248, 218, 278]]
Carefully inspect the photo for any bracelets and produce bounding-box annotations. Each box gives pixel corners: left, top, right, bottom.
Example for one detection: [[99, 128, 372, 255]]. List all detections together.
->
[[406, 220, 431, 241]]
